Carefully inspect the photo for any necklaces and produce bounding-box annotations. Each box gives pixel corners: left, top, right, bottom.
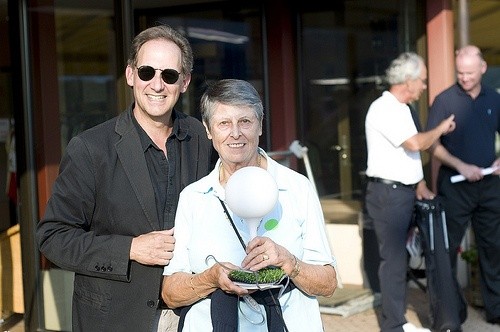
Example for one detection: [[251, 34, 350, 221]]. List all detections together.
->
[[219, 155, 261, 181]]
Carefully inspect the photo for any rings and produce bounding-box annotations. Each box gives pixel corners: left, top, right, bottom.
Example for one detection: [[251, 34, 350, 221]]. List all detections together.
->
[[262, 253, 269, 261]]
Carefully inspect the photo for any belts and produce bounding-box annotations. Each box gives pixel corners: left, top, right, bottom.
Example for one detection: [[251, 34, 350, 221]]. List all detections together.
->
[[157, 296, 180, 311], [365, 172, 416, 190]]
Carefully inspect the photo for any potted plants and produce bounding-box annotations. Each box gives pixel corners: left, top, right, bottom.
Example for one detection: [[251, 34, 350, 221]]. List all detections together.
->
[[460, 246, 484, 308]]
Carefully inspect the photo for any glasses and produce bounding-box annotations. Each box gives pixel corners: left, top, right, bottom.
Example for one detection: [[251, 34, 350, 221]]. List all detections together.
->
[[136, 65, 184, 84]]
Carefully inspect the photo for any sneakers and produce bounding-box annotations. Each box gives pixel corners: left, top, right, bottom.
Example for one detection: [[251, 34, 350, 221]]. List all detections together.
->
[[391, 320, 432, 332]]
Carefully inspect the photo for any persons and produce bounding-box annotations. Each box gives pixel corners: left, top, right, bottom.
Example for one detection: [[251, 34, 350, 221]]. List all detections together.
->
[[35, 25, 219, 332], [365, 52, 454, 332], [162, 79, 338, 332], [426, 45, 500, 332]]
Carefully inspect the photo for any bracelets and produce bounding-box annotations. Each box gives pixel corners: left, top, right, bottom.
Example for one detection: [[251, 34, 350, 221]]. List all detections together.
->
[[190, 275, 215, 298], [288, 255, 300, 279]]
